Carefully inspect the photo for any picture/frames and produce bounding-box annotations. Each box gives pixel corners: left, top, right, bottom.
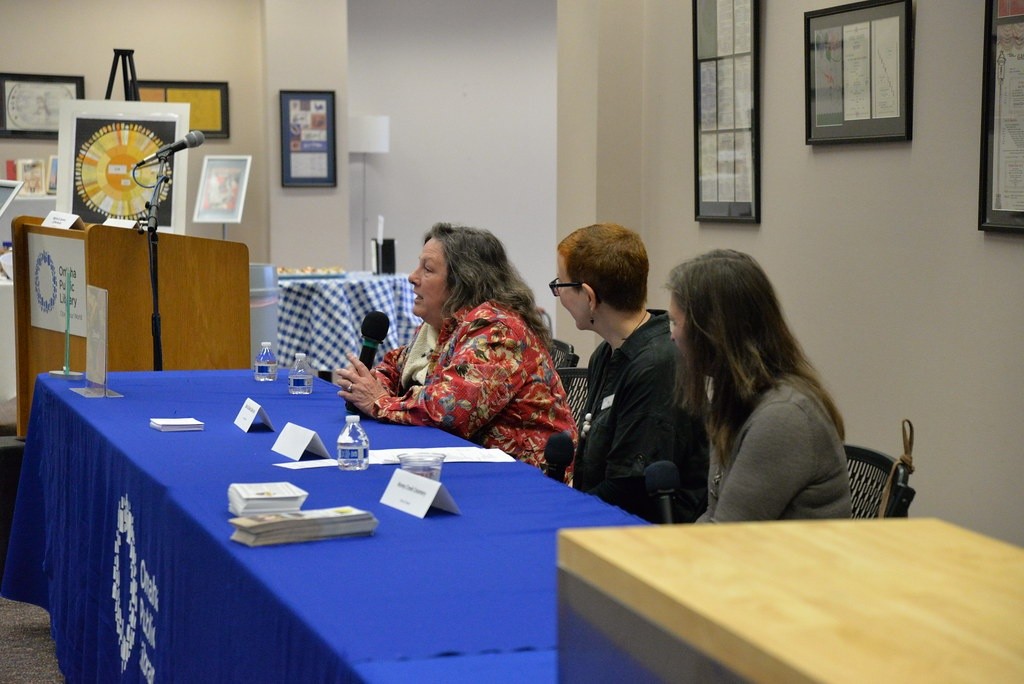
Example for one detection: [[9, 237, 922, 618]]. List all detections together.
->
[[691, 0, 757, 221], [0, 74, 84, 137], [804, 1, 913, 144], [131, 79, 230, 140], [977, 1, 1024, 230], [282, 91, 335, 190]]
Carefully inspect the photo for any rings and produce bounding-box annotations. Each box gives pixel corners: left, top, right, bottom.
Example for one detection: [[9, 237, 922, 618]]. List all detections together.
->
[[347, 383, 353, 392]]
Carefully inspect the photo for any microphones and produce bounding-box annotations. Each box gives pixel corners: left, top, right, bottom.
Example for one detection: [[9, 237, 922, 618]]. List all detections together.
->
[[136, 130, 205, 167], [346, 311, 389, 412], [645, 460, 680, 524], [544, 434, 574, 483]]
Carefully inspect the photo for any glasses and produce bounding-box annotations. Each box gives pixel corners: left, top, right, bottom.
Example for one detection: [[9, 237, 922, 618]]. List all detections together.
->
[[548, 277, 602, 304]]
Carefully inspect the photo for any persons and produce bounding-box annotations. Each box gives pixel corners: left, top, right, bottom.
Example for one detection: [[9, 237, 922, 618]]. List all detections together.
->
[[663, 248, 852, 524], [550, 221, 709, 525], [336, 221, 579, 492]]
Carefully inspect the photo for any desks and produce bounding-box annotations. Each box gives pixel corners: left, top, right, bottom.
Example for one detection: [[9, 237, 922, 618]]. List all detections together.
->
[[0, 365, 655, 683], [277, 269, 425, 379]]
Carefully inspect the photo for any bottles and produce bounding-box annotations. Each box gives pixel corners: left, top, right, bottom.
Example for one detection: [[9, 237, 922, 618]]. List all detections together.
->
[[254, 342, 277, 382], [337, 415, 370, 470], [288, 353, 314, 395], [0, 242, 12, 278]]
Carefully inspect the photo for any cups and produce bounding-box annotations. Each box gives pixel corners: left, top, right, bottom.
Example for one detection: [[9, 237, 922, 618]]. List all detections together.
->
[[397, 452, 446, 484]]
